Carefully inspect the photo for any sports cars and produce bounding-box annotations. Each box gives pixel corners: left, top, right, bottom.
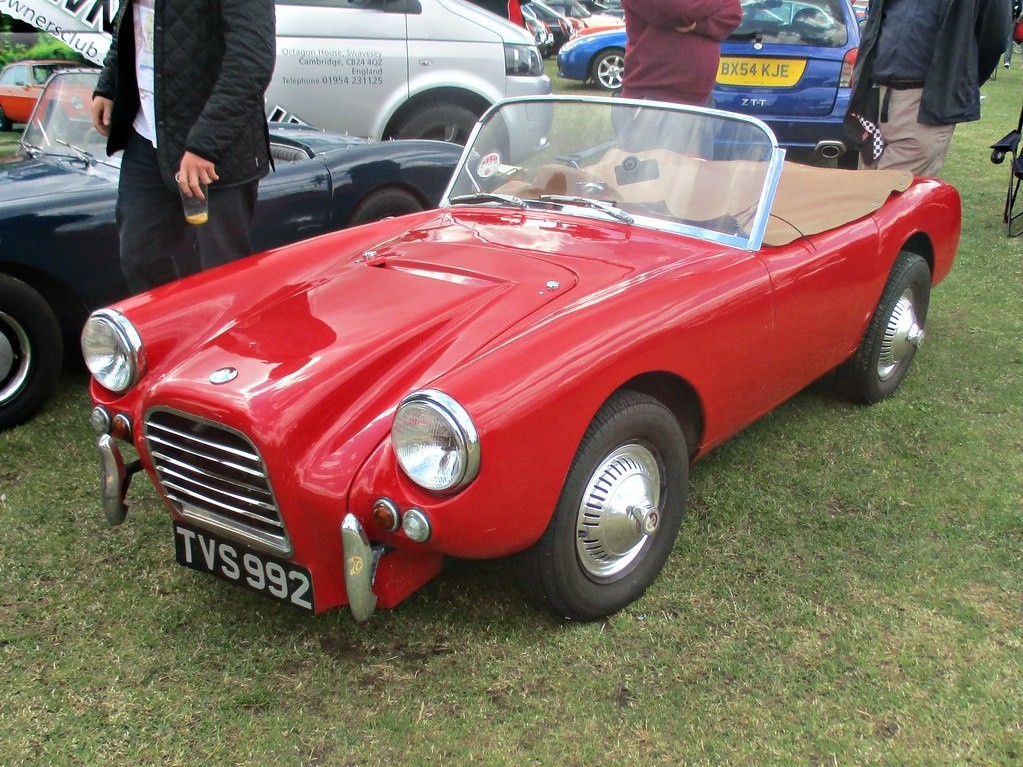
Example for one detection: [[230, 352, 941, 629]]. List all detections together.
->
[[80, 92, 963, 620], [0, 69, 485, 430]]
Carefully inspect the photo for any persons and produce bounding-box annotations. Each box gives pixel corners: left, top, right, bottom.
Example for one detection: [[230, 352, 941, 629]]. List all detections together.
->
[[845, 0, 1014, 188], [92, 0, 275, 294], [620, 0, 743, 165]]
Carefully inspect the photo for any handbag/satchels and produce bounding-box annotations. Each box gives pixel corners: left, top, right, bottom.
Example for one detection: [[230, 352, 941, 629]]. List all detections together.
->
[[1013, 20, 1023, 44]]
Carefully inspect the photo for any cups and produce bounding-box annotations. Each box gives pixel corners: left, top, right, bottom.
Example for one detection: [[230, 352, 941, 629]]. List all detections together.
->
[[175, 170, 210, 225]]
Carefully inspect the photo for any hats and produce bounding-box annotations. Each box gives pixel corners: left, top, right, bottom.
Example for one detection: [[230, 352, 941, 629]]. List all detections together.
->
[[840, 84, 884, 164]]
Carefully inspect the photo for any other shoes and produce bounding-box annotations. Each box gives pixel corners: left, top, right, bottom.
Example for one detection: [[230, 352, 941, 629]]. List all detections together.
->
[[1004, 63, 1010, 69]]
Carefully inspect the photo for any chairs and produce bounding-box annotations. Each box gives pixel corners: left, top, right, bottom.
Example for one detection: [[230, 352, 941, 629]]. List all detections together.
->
[[989, 105, 1023, 237], [792, 8, 835, 47]]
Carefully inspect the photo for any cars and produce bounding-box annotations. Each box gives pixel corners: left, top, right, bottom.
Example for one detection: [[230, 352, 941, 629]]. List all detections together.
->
[[720, 0, 867, 160], [474, 1, 631, 92], [0, 58, 100, 129]]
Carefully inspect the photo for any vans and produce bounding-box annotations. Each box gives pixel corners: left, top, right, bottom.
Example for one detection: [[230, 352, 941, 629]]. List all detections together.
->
[[257, 0, 555, 171]]
